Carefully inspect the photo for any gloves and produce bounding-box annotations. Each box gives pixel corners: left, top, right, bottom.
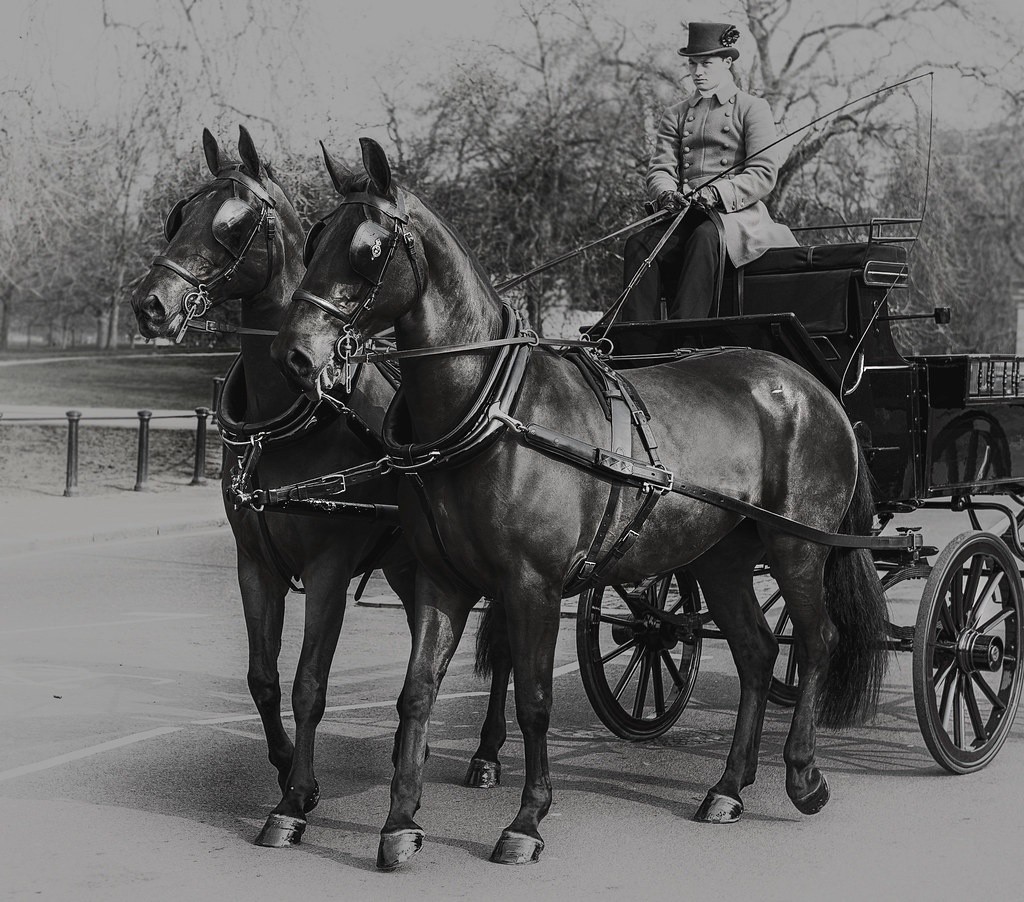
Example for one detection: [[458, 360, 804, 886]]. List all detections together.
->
[[657, 190, 690, 214], [689, 185, 721, 212]]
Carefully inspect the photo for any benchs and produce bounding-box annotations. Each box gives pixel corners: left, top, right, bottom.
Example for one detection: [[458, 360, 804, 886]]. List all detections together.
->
[[658, 217, 919, 492]]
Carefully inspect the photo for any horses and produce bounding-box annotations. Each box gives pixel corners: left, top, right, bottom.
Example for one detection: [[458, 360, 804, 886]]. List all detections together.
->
[[131, 118, 894, 873]]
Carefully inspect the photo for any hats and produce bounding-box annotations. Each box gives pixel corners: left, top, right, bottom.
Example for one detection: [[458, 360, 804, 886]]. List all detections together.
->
[[678, 23, 741, 64]]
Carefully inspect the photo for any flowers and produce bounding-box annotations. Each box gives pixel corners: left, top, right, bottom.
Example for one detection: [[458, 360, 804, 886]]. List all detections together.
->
[[722, 25, 740, 48]]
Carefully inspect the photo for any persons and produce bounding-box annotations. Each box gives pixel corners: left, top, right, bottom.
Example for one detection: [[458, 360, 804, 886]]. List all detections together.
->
[[622, 20, 778, 322]]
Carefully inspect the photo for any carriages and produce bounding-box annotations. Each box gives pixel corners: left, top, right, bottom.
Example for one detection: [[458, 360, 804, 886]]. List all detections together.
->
[[130, 121, 1023, 872]]
